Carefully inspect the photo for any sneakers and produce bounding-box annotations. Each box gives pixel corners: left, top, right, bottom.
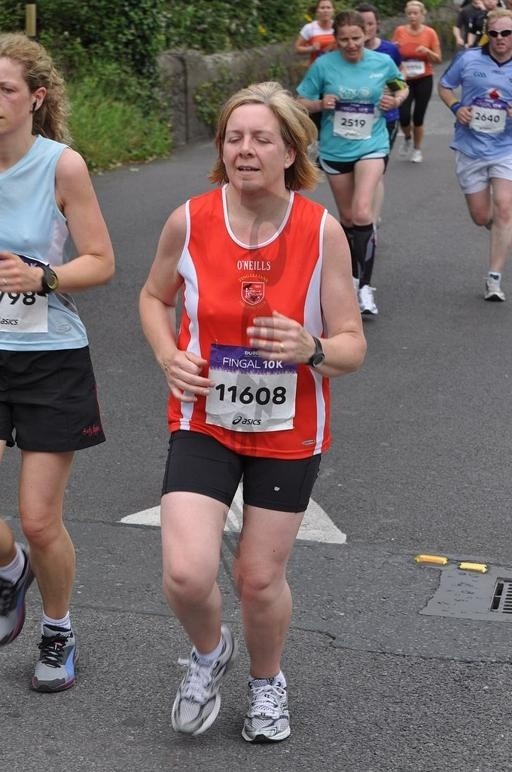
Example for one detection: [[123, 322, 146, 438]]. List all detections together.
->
[[398, 141, 410, 158], [1, 543, 37, 644], [409, 149, 423, 163], [240, 673, 291, 743], [170, 626, 239, 736], [357, 286, 379, 315], [481, 272, 505, 303], [29, 622, 78, 693]]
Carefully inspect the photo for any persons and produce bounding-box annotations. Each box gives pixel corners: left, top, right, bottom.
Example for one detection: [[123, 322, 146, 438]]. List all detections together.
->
[[292, 9, 414, 318], [451, 0, 507, 49], [0, 32, 117, 693], [295, 1, 443, 231], [134, 79, 370, 746], [436, 9, 510, 303]]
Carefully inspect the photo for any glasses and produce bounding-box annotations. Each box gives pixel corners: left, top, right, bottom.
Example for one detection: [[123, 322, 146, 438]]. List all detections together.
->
[[488, 30, 511, 37]]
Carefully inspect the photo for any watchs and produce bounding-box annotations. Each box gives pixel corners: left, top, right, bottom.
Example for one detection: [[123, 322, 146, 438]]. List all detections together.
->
[[36, 263, 61, 298], [307, 336, 326, 372]]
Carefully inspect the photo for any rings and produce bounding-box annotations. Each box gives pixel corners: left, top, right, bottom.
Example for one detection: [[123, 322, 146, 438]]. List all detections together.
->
[[280, 343, 285, 352], [3, 278, 9, 286]]
